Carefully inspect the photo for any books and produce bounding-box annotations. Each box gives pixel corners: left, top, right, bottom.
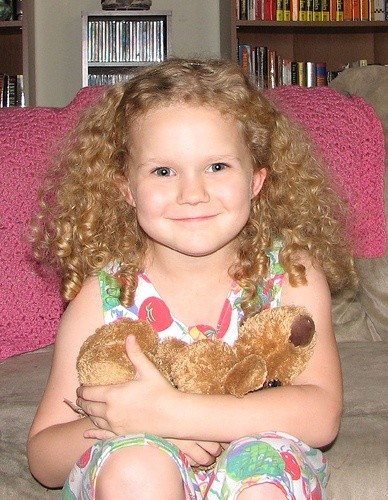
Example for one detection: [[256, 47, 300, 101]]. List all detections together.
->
[[86, 73, 134, 86], [239, 44, 341, 91], [0, 73, 25, 108], [236, 0, 388, 22], [87, 21, 164, 62]]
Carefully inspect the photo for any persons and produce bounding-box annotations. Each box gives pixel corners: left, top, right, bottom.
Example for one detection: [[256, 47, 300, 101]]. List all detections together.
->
[[25, 60, 362, 500]]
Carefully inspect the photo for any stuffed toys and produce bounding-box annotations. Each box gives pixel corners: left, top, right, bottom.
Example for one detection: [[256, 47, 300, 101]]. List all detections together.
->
[[76, 305, 318, 398]]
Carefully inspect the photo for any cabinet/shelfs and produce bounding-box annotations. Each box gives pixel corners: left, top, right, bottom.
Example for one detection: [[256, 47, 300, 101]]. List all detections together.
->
[[81, 9, 172, 88], [220, 0, 388, 91], [0, 0, 30, 108]]
